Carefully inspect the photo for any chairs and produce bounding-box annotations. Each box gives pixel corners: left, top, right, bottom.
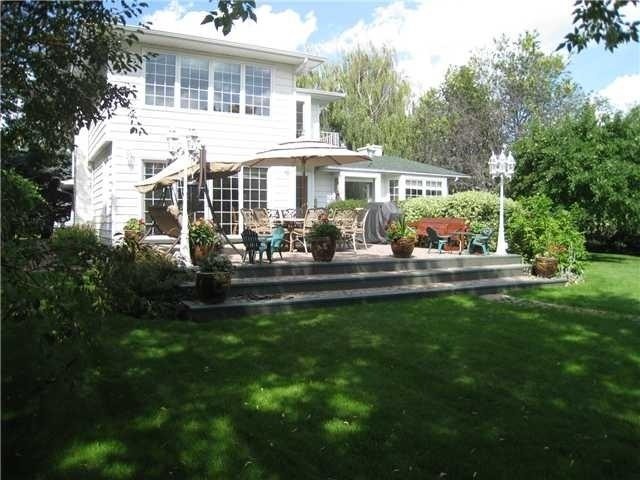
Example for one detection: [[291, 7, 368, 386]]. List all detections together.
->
[[469, 228, 493, 255], [426, 227, 449, 254], [241, 207, 370, 264]]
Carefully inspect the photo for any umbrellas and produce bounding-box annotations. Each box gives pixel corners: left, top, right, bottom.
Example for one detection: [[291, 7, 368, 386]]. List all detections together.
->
[[132, 151, 248, 262], [243, 130, 375, 219]]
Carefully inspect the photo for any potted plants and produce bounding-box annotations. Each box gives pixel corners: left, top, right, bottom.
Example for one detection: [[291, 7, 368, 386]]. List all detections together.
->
[[189, 218, 225, 265], [124, 217, 146, 243], [195, 251, 233, 304], [531, 246, 559, 278], [385, 214, 419, 258]]
